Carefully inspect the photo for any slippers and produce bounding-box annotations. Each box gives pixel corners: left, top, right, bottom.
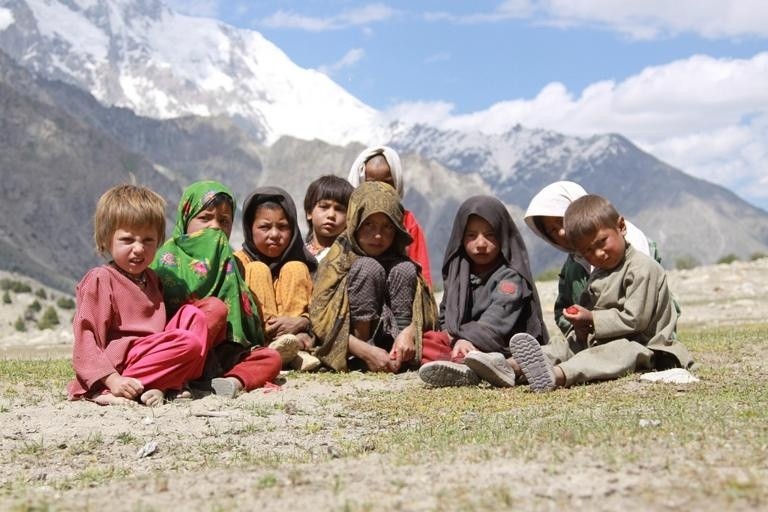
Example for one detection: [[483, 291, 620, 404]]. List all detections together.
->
[[170, 382, 195, 403], [194, 377, 236, 399], [267, 333, 321, 372]]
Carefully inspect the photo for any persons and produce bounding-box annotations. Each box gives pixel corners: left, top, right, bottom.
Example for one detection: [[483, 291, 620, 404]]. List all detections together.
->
[[464, 194, 694, 391], [66, 184, 208, 407], [419, 195, 548, 387], [148, 180, 282, 398], [524, 180, 658, 337]]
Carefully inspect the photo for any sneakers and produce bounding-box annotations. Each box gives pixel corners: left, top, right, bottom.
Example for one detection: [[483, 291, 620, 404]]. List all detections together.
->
[[508, 332, 558, 394], [464, 350, 516, 388], [419, 359, 480, 387]]
